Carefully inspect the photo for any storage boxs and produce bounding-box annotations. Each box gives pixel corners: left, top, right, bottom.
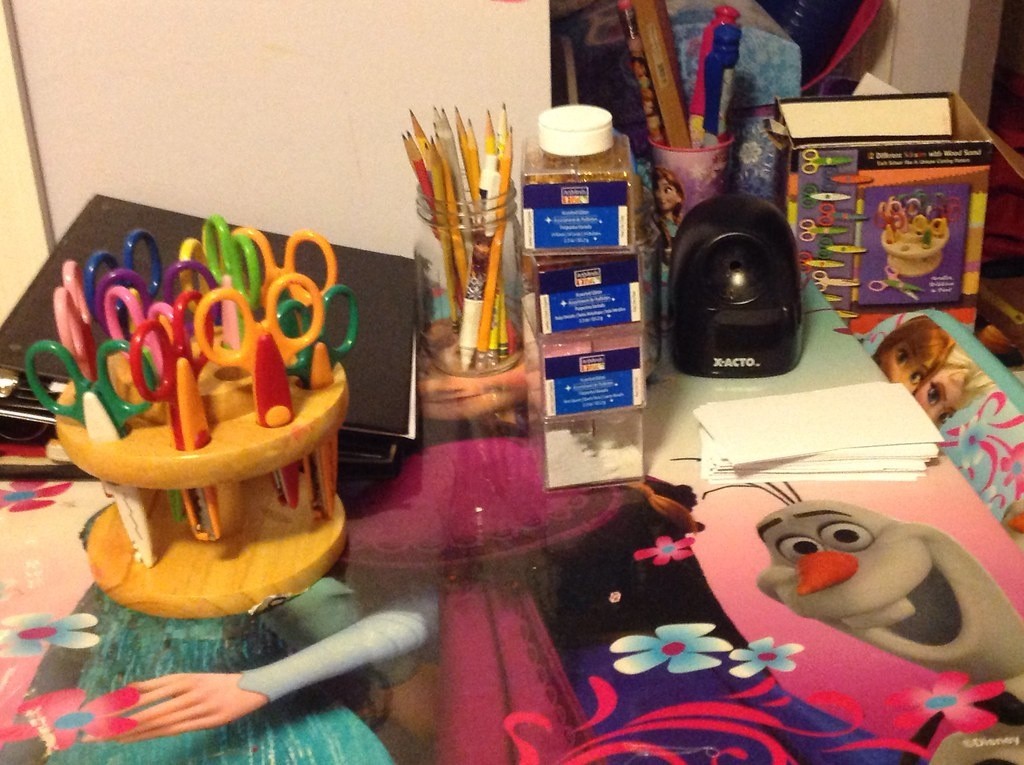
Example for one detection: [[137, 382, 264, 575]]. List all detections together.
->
[[764, 88, 1024, 308]]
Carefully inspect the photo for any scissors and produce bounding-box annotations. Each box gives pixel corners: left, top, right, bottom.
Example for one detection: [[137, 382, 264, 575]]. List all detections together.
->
[[24, 211, 360, 569], [796, 148, 874, 319]]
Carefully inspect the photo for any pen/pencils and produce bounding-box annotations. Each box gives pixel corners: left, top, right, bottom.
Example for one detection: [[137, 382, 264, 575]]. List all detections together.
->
[[617, 0, 742, 148], [401, 100, 515, 370]]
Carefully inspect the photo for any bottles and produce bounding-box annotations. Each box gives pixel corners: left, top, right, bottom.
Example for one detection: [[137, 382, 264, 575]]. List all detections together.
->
[[414, 178, 524, 376]]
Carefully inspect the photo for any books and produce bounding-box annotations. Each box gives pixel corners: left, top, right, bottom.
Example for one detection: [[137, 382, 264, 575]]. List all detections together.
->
[[0, 194, 421, 517]]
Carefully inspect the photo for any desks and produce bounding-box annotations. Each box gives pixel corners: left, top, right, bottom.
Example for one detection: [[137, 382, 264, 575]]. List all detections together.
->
[[0, 283, 1024, 765]]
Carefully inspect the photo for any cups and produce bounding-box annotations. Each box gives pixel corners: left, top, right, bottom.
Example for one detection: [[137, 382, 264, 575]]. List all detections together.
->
[[650, 131, 735, 246]]
